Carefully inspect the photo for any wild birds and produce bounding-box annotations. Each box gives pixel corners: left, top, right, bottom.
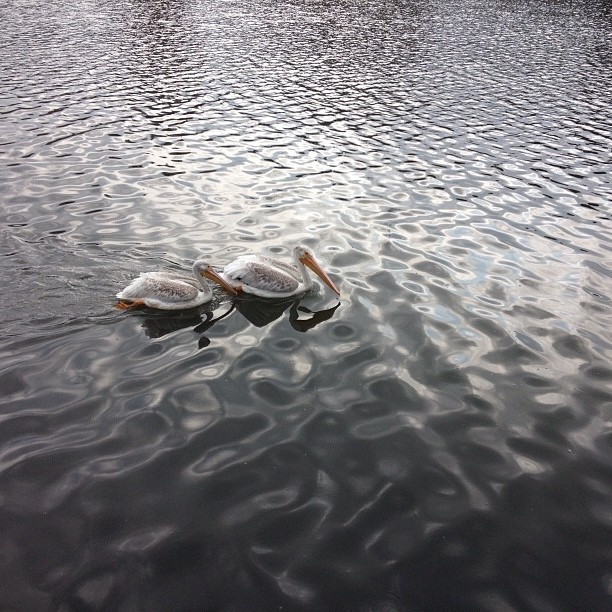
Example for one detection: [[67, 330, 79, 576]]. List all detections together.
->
[[221, 246, 340, 299], [116, 259, 238, 311]]
